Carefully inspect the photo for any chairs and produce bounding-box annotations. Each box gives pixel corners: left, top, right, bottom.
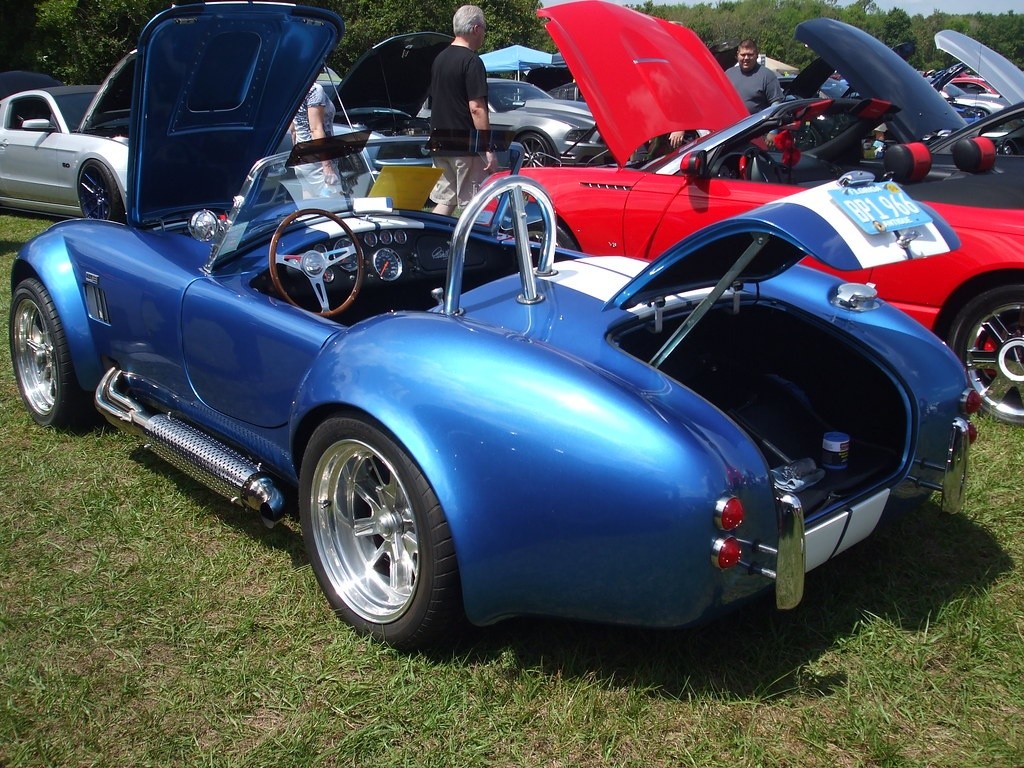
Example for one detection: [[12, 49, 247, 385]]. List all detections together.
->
[[942, 136, 997, 181], [882, 142, 932, 185]]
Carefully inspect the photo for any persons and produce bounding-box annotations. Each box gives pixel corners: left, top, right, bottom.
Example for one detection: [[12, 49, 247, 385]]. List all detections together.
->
[[428, 5, 498, 217], [668, 131, 685, 148], [290, 79, 349, 212], [724, 39, 784, 149]]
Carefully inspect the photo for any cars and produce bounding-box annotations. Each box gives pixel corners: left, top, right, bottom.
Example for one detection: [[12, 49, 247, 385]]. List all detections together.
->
[[0, 2, 1022, 656]]
